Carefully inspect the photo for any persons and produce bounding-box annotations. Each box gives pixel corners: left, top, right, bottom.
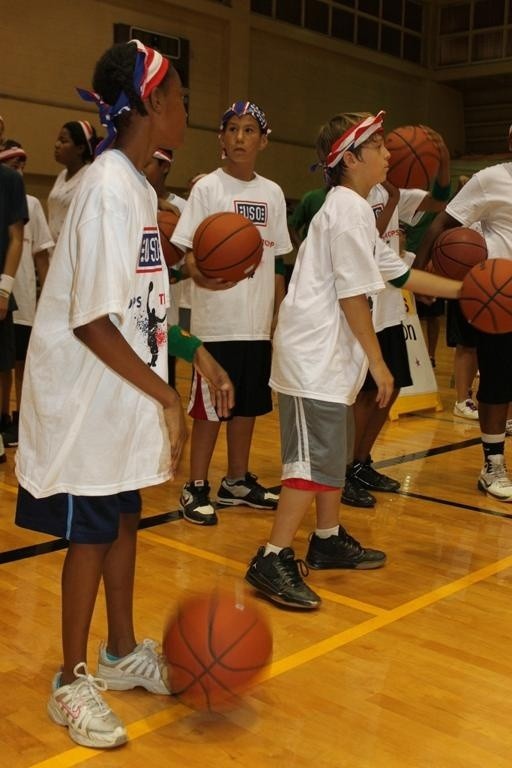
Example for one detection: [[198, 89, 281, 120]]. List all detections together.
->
[[143, 148, 328, 393], [1, 121, 102, 461], [168, 101, 294, 525], [342, 144, 511, 506], [243, 108, 464, 611], [14, 36, 237, 750]]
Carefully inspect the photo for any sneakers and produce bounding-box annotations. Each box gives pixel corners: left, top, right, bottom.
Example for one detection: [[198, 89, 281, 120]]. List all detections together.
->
[[341, 454, 400, 507], [179, 480, 218, 526], [98, 639, 170, 696], [0, 411, 19, 464], [46, 662, 127, 748], [216, 472, 279, 510], [305, 526, 385, 569], [453, 399, 512, 501], [244, 545, 321, 609]]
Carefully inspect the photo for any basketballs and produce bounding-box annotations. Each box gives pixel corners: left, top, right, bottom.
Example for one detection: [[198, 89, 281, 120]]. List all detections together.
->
[[431, 226, 487, 281], [157, 210, 185, 266], [459, 258, 512, 334], [163, 592, 274, 718], [382, 126, 445, 190], [193, 211, 263, 283]]
